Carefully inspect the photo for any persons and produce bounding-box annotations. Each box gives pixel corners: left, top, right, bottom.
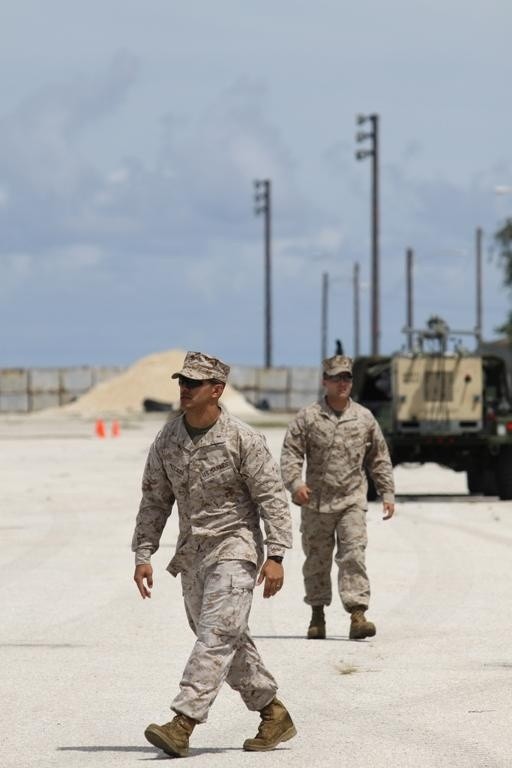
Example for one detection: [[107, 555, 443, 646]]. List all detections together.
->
[[133, 349, 297, 757], [279, 355, 396, 640]]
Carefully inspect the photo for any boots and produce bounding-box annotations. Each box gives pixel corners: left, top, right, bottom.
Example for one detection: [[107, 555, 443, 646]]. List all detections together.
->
[[243, 698, 297, 751], [145, 714, 196, 757], [307, 605, 326, 639], [349, 607, 376, 638]]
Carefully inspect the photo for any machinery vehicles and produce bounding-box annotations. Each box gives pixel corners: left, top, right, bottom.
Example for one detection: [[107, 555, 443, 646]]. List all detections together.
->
[[348, 310, 511, 504]]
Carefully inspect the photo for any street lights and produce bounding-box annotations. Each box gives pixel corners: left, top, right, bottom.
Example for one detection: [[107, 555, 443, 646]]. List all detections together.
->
[[351, 108, 386, 357], [250, 175, 274, 369], [493, 184, 512, 195]]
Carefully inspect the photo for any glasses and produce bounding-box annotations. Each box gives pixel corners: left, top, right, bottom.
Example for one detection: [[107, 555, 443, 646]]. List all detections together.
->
[[332, 374, 353, 382], [179, 376, 215, 388]]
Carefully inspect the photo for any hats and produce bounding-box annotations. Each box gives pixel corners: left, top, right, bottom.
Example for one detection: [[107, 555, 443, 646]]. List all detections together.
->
[[171, 352, 229, 383], [322, 356, 353, 376]]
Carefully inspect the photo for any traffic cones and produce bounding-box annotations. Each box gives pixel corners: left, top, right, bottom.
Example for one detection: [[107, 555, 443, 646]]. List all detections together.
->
[[108, 418, 120, 438], [94, 418, 105, 438]]
